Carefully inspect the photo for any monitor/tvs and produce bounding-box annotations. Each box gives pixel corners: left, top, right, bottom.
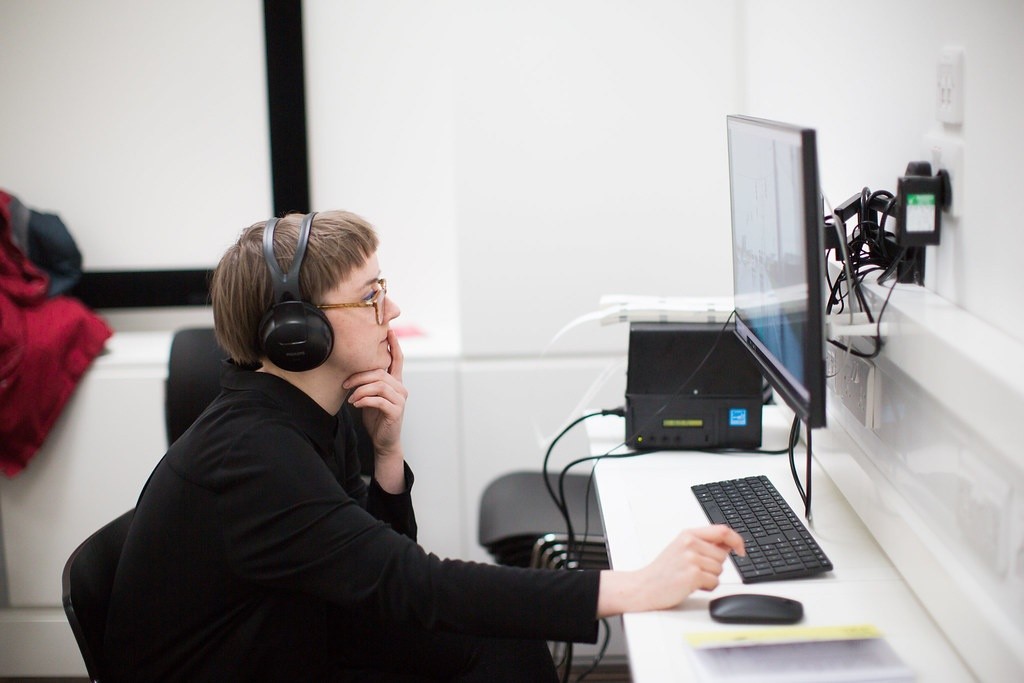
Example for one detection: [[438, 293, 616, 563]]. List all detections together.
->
[[725, 114, 825, 429]]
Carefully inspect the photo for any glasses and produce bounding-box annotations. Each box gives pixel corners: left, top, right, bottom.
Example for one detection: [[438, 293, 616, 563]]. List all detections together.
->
[[316, 278, 388, 326]]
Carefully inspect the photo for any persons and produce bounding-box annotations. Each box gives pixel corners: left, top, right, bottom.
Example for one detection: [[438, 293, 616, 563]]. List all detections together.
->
[[105, 210, 746, 683]]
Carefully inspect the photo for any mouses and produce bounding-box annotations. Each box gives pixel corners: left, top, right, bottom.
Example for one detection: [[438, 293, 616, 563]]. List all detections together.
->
[[709, 594, 802, 624]]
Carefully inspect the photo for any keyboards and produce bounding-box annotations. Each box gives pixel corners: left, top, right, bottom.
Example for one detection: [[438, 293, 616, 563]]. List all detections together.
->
[[691, 476, 833, 584]]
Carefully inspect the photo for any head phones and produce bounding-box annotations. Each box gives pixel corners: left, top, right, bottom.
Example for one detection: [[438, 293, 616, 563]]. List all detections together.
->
[[256, 212, 334, 372]]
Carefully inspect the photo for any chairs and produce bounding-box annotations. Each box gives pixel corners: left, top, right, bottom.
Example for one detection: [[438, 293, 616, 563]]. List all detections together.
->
[[61, 506, 136, 683], [478, 470, 613, 571]]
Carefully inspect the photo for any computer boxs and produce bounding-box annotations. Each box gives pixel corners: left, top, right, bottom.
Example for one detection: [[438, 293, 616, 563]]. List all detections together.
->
[[624, 323, 765, 450]]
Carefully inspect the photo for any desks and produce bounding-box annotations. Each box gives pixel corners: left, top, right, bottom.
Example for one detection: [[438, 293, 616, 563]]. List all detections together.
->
[[583, 407, 981, 683]]
